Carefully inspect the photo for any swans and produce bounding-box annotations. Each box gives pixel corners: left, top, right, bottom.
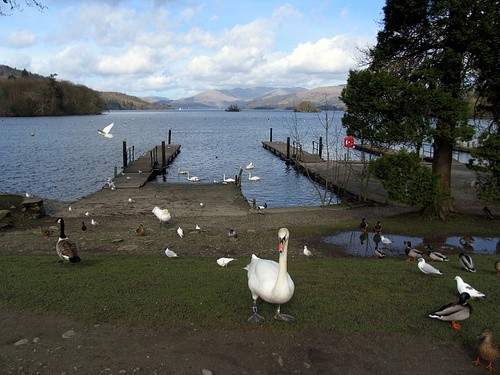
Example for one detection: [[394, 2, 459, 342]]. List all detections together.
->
[[245, 161, 254, 170], [222, 173, 236, 182], [243, 227, 295, 325], [175, 167, 201, 182], [248, 171, 260, 181]]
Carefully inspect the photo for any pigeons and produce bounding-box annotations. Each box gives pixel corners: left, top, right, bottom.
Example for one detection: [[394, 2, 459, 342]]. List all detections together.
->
[[417, 257, 444, 276], [380, 235, 395, 245], [195, 223, 201, 230], [303, 245, 316, 257], [90, 217, 101, 226], [454, 275, 486, 300], [216, 257, 238, 268], [164, 247, 180, 259], [176, 226, 185, 239], [25, 192, 36, 200], [81, 221, 87, 231]]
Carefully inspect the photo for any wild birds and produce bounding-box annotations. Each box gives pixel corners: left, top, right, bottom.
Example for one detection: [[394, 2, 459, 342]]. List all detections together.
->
[[97, 122, 115, 140], [135, 224, 145, 236]]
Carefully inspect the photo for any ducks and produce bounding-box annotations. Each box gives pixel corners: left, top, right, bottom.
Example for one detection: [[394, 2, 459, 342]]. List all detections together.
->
[[54, 218, 82, 267], [403, 240, 425, 263], [426, 248, 449, 262], [426, 292, 473, 330], [471, 329, 500, 373], [374, 220, 382, 234], [374, 246, 386, 259], [359, 233, 369, 245], [358, 216, 369, 233], [456, 252, 476, 273], [39, 225, 54, 238], [372, 234, 381, 246]]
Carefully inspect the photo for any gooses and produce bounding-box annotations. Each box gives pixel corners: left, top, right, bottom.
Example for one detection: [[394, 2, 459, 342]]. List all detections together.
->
[[152, 205, 171, 226]]
